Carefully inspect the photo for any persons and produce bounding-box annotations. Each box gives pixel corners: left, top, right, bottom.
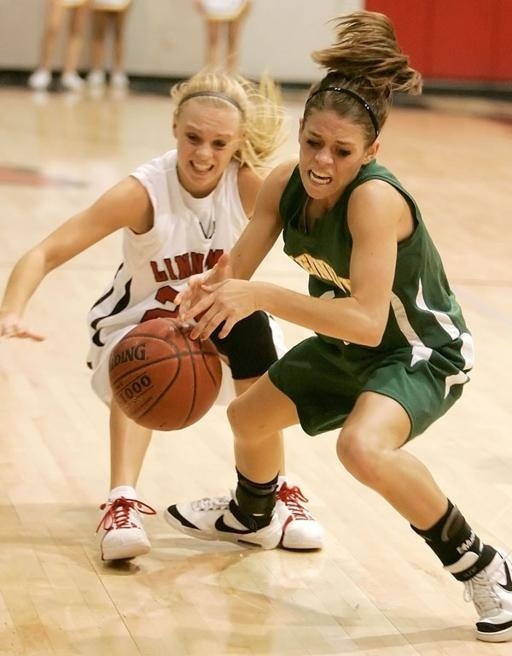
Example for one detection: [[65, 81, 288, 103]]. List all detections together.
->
[[84, 1, 134, 91], [194, 1, 250, 72], [171, 11, 512, 645], [0, 64, 322, 564], [27, 1, 94, 92]]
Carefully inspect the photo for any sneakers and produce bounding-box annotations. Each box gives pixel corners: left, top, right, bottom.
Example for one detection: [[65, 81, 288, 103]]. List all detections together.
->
[[96, 494, 157, 559], [161, 490, 283, 551], [442, 546, 512, 644], [275, 482, 321, 551]]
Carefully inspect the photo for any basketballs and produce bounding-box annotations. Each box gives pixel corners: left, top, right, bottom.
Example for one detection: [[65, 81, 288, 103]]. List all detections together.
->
[[106, 316, 223, 432]]
